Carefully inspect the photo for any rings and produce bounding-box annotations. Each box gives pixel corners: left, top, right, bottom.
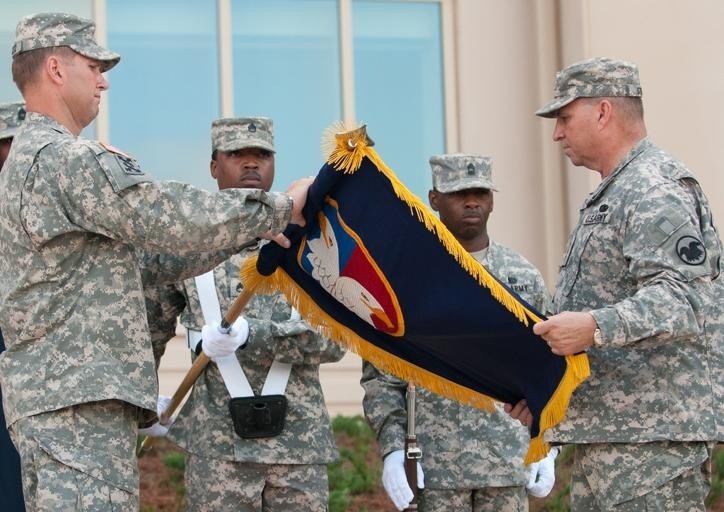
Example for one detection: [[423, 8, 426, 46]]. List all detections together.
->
[[548, 340, 552, 348]]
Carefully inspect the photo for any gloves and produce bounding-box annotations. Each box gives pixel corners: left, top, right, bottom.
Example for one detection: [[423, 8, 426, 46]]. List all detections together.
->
[[201, 316, 249, 358], [381, 449, 425, 511], [139, 395, 177, 439], [526, 446, 559, 499]]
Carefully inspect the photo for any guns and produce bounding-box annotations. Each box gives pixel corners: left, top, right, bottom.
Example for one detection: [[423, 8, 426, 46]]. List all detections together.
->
[[405, 378, 422, 512]]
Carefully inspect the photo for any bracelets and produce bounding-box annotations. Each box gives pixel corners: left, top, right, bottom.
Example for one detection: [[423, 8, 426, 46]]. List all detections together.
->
[[284, 194, 294, 211]]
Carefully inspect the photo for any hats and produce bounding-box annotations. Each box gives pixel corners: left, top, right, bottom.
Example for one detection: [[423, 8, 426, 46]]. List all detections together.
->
[[11, 11, 122, 72], [429, 155, 499, 195], [535, 57, 642, 119], [211, 118, 276, 155], [0, 101, 25, 140]]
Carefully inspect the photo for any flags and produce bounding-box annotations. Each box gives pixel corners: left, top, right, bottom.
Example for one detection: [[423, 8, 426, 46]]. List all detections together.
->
[[240, 127, 590, 466]]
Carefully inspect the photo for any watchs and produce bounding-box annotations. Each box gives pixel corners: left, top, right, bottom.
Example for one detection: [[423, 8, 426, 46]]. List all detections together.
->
[[594, 322, 603, 348]]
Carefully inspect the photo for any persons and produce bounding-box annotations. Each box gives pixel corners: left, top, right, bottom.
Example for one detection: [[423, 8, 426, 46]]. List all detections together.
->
[[0, 100, 26, 166], [0, 11, 315, 512], [503, 58, 724, 511], [138, 115, 351, 511], [360, 153, 563, 511]]
